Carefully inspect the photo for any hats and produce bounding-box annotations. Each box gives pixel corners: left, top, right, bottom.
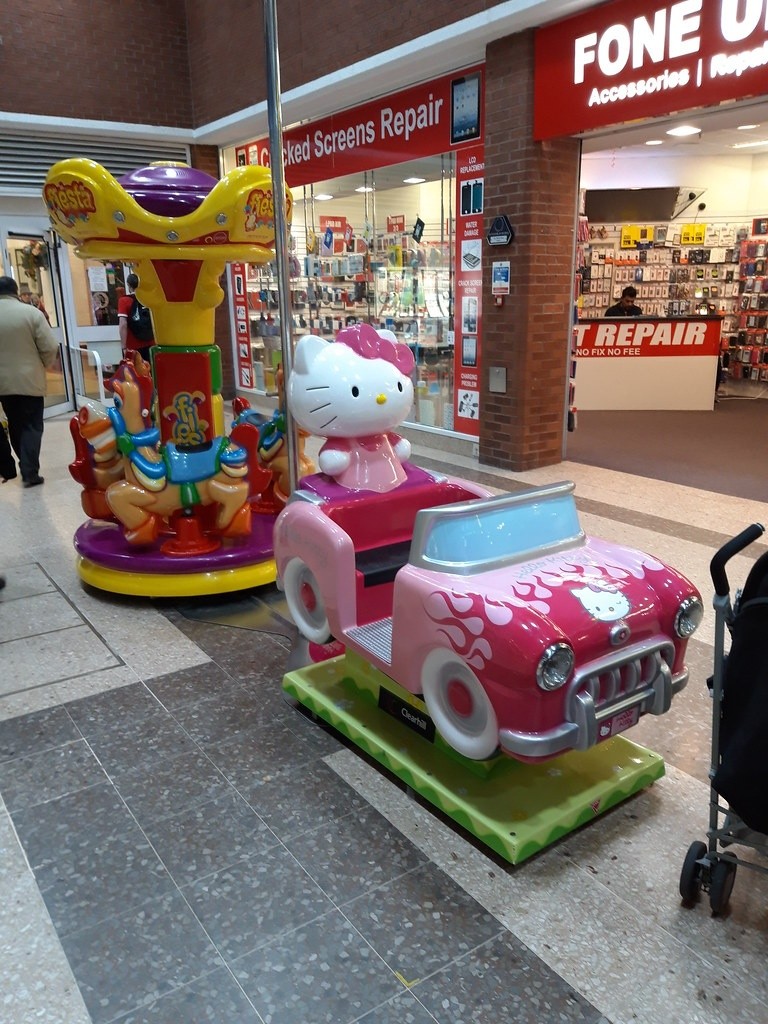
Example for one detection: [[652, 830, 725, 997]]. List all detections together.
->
[[19, 286, 33, 297]]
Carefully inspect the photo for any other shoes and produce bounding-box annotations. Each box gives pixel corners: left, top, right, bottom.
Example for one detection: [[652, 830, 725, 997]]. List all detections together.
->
[[24, 477, 44, 487], [0, 578, 6, 590]]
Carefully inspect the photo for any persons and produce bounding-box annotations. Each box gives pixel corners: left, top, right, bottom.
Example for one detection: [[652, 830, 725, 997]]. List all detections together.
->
[[0, 276, 58, 489], [604, 286, 643, 316], [118, 274, 157, 420]]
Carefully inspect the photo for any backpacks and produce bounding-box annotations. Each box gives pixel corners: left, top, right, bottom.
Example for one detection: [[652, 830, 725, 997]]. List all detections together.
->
[[125, 294, 154, 343]]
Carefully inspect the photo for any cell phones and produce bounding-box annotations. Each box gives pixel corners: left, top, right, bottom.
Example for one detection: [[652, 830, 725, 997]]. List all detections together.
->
[[467, 297, 477, 332], [473, 183, 482, 213], [462, 185, 471, 214]]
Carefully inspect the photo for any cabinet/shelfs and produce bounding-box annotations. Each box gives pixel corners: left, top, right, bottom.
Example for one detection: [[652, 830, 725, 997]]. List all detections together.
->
[[246, 223, 458, 386], [566, 221, 768, 433]]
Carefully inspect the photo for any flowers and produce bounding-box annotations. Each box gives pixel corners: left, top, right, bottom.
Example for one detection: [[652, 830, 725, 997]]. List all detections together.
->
[[21, 239, 49, 280]]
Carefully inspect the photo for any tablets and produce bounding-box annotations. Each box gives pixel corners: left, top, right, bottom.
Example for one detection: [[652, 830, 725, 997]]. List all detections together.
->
[[450, 70, 481, 145], [461, 336, 478, 369], [463, 253, 480, 267]]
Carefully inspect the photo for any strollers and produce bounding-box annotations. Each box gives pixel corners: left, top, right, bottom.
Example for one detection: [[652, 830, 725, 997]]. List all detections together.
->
[[678, 522, 768, 922]]
